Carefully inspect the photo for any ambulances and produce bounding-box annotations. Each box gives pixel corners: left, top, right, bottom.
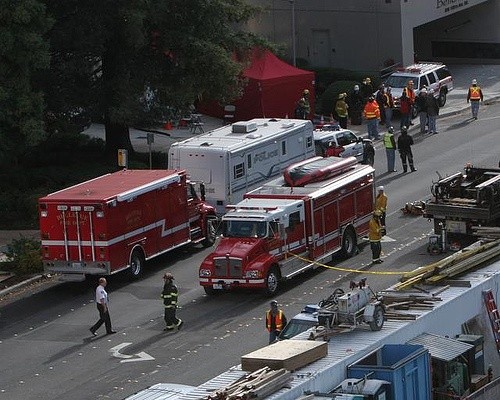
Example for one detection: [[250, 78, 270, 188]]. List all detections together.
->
[[37, 169, 219, 283]]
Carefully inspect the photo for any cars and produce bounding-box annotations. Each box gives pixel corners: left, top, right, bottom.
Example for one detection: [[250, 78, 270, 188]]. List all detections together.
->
[[271, 303, 320, 344]]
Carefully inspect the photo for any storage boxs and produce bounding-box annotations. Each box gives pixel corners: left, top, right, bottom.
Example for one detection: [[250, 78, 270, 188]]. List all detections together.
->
[[240, 339, 328, 372]]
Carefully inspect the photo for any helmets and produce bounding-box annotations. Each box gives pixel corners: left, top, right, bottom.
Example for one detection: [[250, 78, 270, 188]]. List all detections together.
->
[[400, 126, 407, 133], [337, 92, 347, 100], [304, 89, 310, 95], [430, 89, 435, 94], [389, 127, 393, 132], [472, 79, 477, 85], [408, 80, 414, 85], [366, 78, 371, 83], [354, 85, 359, 90], [420, 88, 427, 93], [163, 273, 174, 279]]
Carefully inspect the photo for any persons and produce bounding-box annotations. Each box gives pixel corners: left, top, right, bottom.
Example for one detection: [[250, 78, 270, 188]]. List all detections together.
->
[[350, 78, 439, 140], [370, 209, 386, 264], [265, 300, 287, 344], [89, 278, 117, 336], [297, 89, 311, 120], [375, 186, 387, 236], [159, 273, 184, 332], [383, 126, 397, 172], [467, 79, 483, 120], [189, 113, 204, 134], [336, 94, 349, 129], [398, 126, 417, 173]]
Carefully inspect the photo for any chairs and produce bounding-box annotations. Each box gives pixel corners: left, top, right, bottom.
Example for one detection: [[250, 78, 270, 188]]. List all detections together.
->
[[224, 104, 238, 125], [191, 114, 205, 135]]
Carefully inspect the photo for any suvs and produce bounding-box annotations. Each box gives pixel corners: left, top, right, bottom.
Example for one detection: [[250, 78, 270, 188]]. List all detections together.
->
[[371, 62, 454, 120]]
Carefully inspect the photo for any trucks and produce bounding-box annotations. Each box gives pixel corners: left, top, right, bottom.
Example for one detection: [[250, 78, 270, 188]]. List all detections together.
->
[[422, 162, 500, 243]]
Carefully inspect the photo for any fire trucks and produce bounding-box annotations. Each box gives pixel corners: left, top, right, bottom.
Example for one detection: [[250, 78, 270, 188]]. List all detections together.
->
[[165, 118, 377, 225], [198, 155, 378, 300]]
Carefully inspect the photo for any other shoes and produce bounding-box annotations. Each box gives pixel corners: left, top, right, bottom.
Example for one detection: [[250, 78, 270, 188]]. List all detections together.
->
[[373, 259, 383, 264], [89, 327, 98, 336], [403, 170, 407, 173], [368, 116, 477, 140], [411, 169, 417, 172], [177, 323, 184, 331], [107, 330, 116, 334], [164, 328, 173, 332], [392, 169, 397, 172]]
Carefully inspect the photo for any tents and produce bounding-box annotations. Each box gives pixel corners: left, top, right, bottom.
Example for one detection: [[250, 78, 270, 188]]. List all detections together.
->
[[193, 44, 316, 122]]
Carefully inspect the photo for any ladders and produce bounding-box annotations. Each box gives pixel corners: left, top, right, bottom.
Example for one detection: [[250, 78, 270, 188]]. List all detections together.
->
[[482, 288, 500, 358]]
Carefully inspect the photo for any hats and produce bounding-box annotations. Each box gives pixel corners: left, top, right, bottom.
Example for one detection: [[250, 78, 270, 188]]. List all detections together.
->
[[271, 300, 277, 307], [371, 209, 383, 218], [378, 186, 385, 190]]
